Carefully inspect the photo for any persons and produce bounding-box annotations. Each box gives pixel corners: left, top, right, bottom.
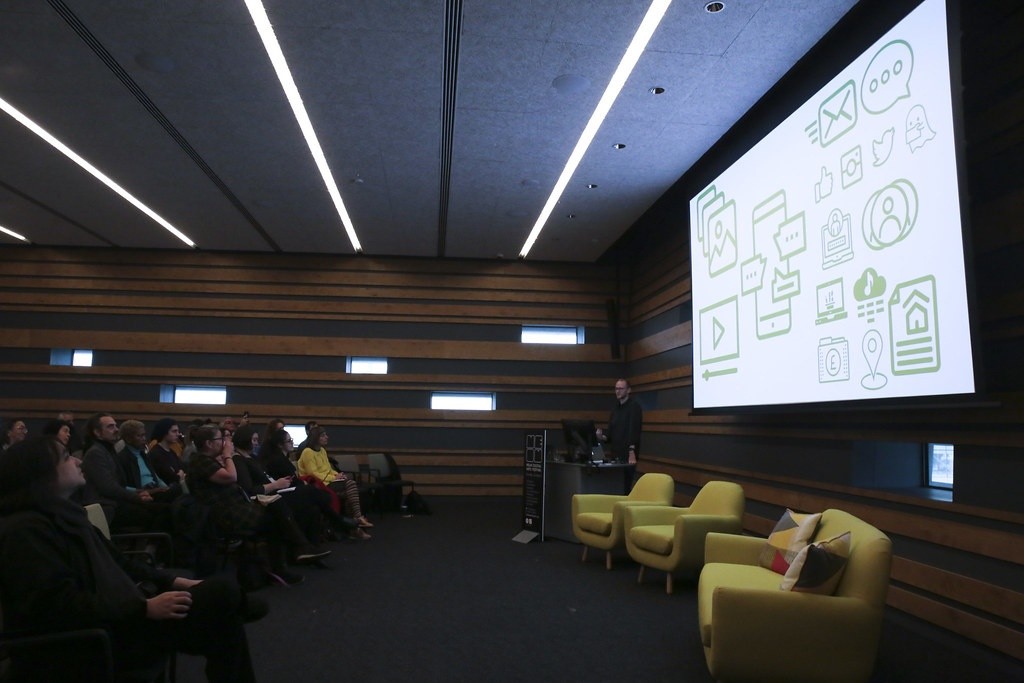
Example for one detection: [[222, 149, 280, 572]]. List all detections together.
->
[[595, 377, 643, 496], [0, 435, 270, 683], [1, 412, 375, 591]]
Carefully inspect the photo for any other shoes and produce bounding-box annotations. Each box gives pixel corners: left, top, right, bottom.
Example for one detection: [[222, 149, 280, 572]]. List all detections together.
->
[[270, 571, 304, 587], [350, 529, 370, 542], [356, 515, 375, 528]]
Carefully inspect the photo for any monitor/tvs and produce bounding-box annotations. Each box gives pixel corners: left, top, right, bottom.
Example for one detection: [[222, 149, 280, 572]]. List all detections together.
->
[[591, 443, 606, 459], [561, 419, 600, 448]]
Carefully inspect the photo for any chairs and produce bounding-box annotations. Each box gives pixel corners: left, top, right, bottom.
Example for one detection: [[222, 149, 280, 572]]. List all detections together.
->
[[365, 452, 418, 517], [332, 453, 381, 516], [570, 471, 676, 571], [623, 479, 746, 595]]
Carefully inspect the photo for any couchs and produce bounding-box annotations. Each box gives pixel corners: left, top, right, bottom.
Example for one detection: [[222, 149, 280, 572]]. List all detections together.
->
[[697, 507, 895, 682]]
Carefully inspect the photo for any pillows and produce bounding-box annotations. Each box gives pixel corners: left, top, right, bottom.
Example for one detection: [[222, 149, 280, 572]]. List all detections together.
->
[[776, 530, 852, 598], [759, 508, 824, 577]]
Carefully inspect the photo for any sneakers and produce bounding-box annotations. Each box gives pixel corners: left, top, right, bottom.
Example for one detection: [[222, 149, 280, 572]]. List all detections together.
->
[[294, 544, 332, 561]]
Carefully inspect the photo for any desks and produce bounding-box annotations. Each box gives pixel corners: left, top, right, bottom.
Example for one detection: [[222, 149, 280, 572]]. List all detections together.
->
[[544, 455, 634, 545]]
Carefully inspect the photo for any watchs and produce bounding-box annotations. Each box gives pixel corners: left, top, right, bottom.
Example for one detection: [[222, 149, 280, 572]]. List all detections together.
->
[[628, 447, 635, 451]]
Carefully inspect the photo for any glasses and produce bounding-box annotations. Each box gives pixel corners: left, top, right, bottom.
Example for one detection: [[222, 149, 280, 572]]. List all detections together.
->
[[213, 436, 225, 441], [283, 438, 294, 444]]
